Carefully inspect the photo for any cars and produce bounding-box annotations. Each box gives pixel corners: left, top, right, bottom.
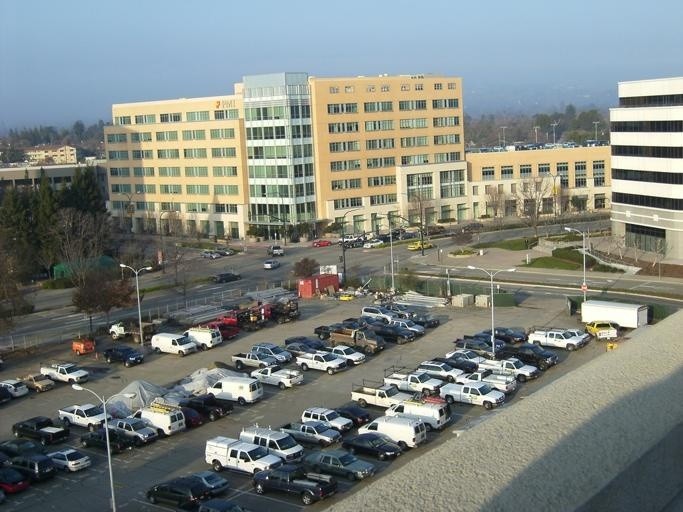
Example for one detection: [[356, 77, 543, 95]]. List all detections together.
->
[[349, 322, 618, 432], [199, 250, 220, 259], [0, 438, 91, 502], [330, 403, 370, 427], [0, 360, 89, 403], [179, 407, 204, 427], [342, 433, 401, 460], [312, 224, 443, 252], [305, 449, 375, 481], [80, 429, 134, 454], [184, 468, 229, 496], [460, 222, 482, 233], [479, 141, 611, 154]]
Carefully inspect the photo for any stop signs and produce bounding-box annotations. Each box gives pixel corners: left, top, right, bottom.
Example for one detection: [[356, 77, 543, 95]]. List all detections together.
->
[[581, 283, 587, 293]]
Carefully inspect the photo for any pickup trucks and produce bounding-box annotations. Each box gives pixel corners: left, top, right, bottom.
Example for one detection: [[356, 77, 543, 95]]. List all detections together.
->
[[203, 436, 284, 477], [280, 420, 341, 446], [11, 416, 69, 447], [249, 461, 338, 504], [251, 366, 304, 389], [103, 417, 158, 446], [57, 403, 112, 432], [179, 391, 232, 420]]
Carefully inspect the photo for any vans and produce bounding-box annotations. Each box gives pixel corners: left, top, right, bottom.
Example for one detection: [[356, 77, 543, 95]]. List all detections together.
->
[[263, 259, 280, 270], [357, 415, 427, 450], [206, 376, 264, 405], [238, 423, 304, 463], [126, 405, 186, 438]]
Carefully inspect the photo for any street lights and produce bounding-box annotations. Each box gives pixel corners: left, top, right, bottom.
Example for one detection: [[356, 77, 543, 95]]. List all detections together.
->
[[492, 120, 600, 145], [400, 192, 424, 255], [159, 210, 179, 273], [374, 214, 395, 295], [341, 206, 364, 289], [465, 265, 517, 357], [563, 227, 588, 302], [117, 190, 140, 232], [118, 261, 152, 347], [546, 172, 563, 223], [264, 192, 287, 247], [71, 381, 136, 512]]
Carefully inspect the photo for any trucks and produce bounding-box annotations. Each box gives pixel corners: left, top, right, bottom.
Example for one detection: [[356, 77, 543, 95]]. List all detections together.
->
[[266, 244, 284, 257]]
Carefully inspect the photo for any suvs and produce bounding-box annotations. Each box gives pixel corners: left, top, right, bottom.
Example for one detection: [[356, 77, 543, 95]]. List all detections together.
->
[[146, 478, 210, 508], [211, 273, 240, 284], [198, 499, 251, 511], [301, 406, 353, 434], [213, 246, 233, 256]]
